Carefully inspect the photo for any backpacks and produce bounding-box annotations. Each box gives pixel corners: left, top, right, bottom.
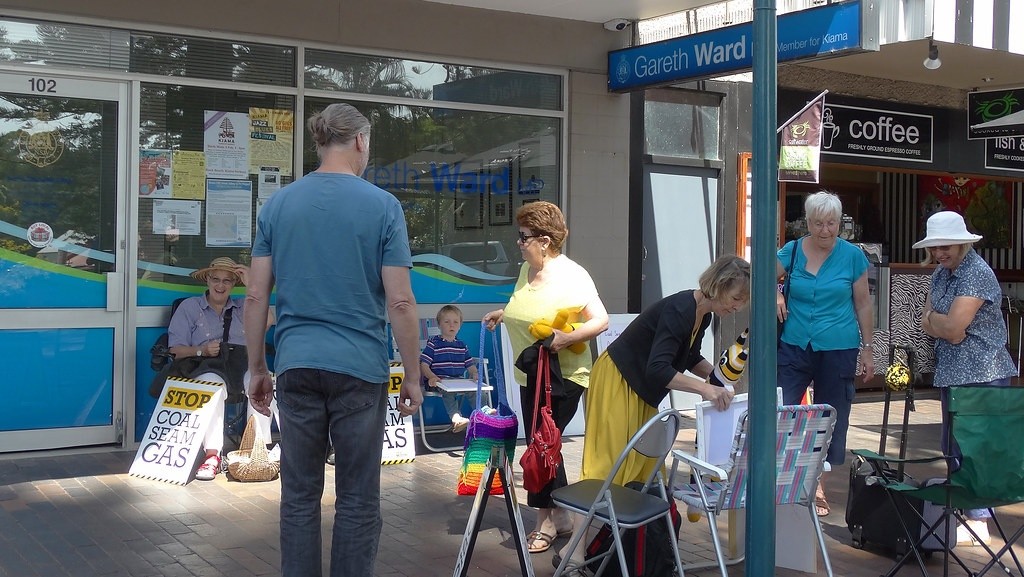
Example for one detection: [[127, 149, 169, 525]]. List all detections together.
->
[[585, 480, 681, 577]]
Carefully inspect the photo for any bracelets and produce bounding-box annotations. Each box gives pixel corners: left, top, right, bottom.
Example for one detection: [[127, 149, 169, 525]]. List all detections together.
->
[[928, 310, 935, 321], [864, 344, 873, 348]]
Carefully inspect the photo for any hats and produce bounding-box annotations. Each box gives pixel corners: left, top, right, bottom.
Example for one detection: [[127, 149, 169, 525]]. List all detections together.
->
[[189, 256, 243, 282], [912, 211, 984, 249]]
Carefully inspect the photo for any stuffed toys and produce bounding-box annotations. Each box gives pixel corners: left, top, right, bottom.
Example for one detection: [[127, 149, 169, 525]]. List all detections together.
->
[[688, 327, 749, 522], [528, 308, 592, 355]]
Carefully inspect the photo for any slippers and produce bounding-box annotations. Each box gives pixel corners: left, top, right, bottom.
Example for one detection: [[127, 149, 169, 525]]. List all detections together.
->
[[815, 488, 829, 516], [528, 531, 558, 552], [556, 529, 573, 537], [485, 408, 498, 416], [553, 555, 587, 577], [453, 418, 470, 433]]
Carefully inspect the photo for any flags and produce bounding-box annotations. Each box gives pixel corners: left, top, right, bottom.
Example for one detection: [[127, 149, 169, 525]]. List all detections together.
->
[[778, 95, 825, 184]]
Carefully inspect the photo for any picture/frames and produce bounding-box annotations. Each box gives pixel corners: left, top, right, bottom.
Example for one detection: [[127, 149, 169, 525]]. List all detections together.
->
[[452, 139, 540, 231]]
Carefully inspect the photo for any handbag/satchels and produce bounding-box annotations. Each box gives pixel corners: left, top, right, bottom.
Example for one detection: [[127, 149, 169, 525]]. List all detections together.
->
[[777, 240, 798, 347], [457, 403, 518, 497], [520, 406, 562, 493], [220, 343, 248, 394]]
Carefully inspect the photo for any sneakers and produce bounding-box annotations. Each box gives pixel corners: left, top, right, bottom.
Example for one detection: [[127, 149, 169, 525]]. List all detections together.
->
[[195, 455, 221, 480], [957, 517, 991, 547]]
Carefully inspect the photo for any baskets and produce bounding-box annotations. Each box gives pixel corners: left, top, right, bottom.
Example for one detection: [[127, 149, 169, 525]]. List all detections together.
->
[[227, 415, 280, 481]]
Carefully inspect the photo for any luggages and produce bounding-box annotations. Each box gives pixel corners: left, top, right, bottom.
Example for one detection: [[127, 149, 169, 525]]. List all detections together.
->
[[846, 345, 958, 562]]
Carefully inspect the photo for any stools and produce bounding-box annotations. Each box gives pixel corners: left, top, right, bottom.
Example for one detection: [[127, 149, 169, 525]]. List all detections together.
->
[[224, 380, 248, 451]]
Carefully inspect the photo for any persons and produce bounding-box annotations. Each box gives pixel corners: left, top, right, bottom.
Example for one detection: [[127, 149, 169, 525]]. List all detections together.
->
[[242, 103, 424, 577], [552, 257, 749, 577], [168, 256, 275, 480], [482, 201, 609, 553], [911, 211, 1019, 546], [420, 305, 497, 433], [776, 189, 875, 517]]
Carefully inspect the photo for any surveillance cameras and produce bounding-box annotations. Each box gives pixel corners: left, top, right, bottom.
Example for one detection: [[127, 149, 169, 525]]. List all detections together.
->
[[603, 19, 627, 32]]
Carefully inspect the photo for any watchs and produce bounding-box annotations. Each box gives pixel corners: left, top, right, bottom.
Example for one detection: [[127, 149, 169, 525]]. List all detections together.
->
[[196, 346, 203, 357]]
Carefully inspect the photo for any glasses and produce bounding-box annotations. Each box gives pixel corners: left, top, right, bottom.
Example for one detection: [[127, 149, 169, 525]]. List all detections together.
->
[[810, 219, 839, 229], [207, 273, 235, 285], [928, 246, 951, 252], [518, 231, 542, 243]]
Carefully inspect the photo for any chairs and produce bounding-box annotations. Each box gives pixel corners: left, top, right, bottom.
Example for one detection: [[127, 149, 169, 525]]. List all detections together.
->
[[848, 386, 1024, 577], [550, 409, 687, 577], [664, 402, 836, 577], [417, 318, 493, 452]]
